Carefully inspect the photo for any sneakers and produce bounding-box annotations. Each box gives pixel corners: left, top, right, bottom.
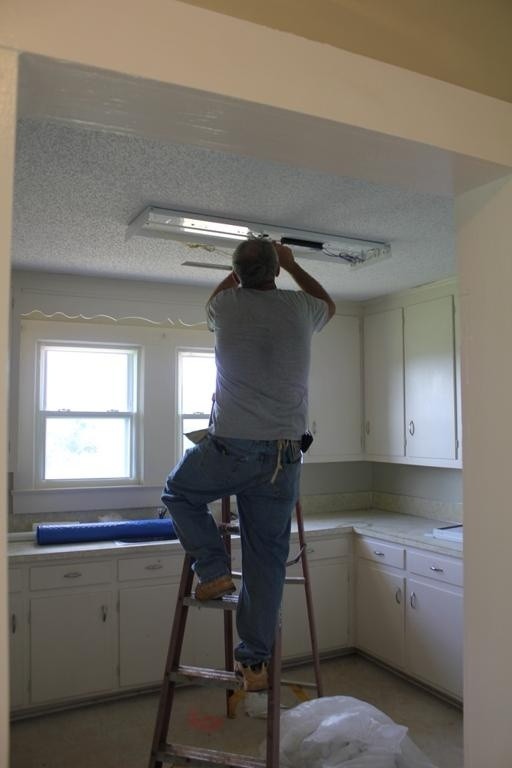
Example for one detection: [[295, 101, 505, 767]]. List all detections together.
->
[[233, 656, 270, 692], [195, 573, 236, 602]]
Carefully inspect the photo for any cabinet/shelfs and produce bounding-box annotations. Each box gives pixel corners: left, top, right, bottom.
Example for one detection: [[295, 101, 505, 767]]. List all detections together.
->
[[281, 538, 351, 663], [360, 292, 404, 465], [118, 553, 242, 691], [351, 534, 464, 711], [11, 560, 29, 714], [29, 559, 117, 716], [302, 302, 363, 464], [405, 276, 463, 470]]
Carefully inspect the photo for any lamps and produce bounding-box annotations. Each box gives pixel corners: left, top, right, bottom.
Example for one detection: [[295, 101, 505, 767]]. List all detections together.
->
[[125, 208, 390, 273]]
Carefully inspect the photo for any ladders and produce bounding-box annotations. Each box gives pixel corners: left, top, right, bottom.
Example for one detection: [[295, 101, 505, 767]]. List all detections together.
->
[[149, 494, 324, 768]]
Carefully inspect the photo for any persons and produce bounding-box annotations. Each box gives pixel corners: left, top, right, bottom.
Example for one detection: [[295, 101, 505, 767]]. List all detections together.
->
[[159, 236, 338, 694]]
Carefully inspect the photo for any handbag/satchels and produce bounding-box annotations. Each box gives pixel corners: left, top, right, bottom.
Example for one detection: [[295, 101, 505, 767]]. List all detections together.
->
[[300, 431, 313, 452]]
